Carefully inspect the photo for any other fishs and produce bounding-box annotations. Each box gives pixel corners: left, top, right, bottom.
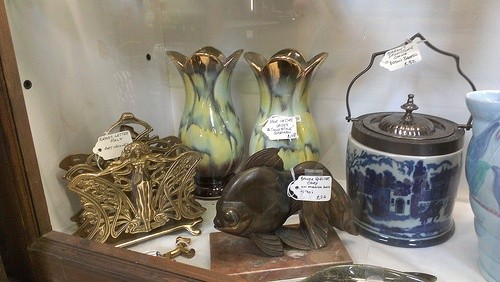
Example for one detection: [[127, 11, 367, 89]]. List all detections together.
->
[[213, 148, 348, 257]]
